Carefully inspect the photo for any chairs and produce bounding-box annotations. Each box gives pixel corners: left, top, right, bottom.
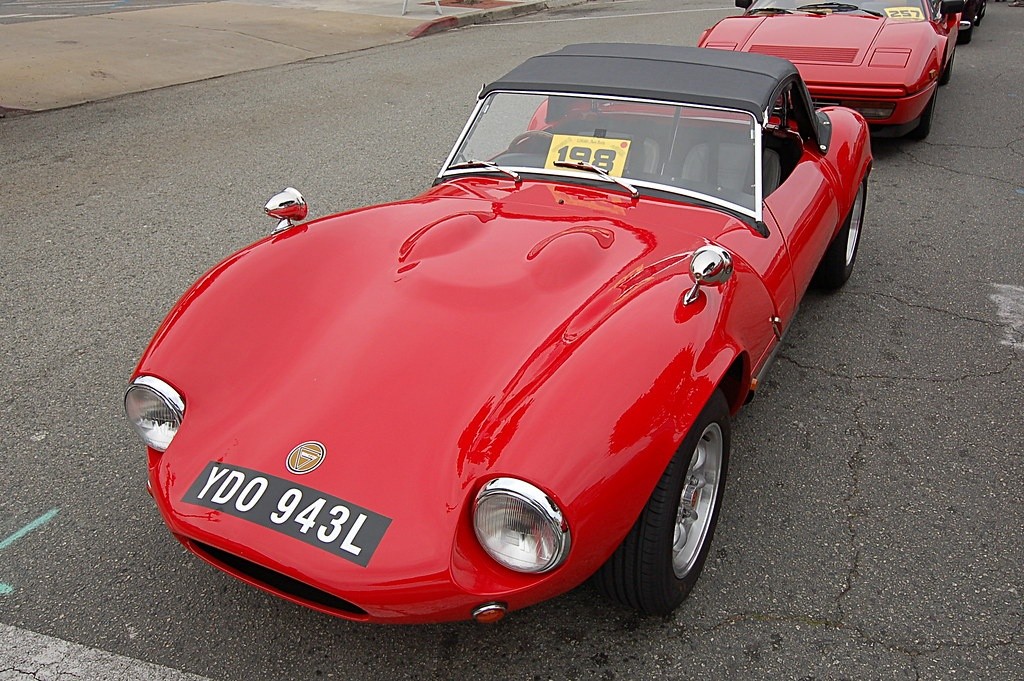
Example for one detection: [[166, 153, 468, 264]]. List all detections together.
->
[[681, 142, 781, 200], [580, 129, 661, 174]]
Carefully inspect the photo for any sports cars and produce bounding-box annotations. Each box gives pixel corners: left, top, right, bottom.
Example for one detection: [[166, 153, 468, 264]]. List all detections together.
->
[[125, 30, 877, 630], [697, 0, 987, 143]]
